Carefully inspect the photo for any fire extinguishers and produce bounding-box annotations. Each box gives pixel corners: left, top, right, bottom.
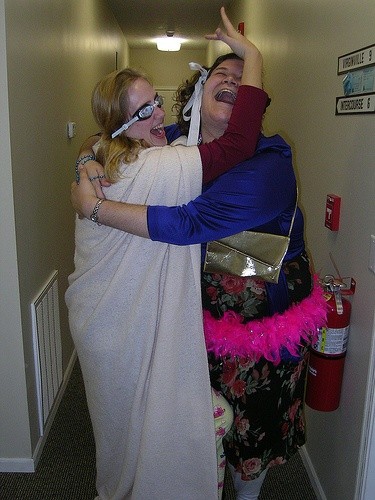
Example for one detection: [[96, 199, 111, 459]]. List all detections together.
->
[[306, 275, 356, 412]]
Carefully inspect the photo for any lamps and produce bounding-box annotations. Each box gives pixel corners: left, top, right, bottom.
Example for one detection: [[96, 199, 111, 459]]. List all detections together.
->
[[156, 30, 183, 51]]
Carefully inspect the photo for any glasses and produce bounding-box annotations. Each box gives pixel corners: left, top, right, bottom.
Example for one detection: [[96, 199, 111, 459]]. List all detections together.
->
[[112, 92, 163, 138]]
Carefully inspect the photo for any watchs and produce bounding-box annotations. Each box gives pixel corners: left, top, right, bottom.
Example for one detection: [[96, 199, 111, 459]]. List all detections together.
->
[[90, 198, 104, 227]]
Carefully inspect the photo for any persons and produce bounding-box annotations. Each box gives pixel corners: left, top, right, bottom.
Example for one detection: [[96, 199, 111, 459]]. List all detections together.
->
[[64, 6, 272, 500], [70, 52, 329, 500]]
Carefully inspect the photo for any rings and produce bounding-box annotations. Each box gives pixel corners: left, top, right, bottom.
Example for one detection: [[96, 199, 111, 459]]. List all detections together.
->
[[99, 175, 106, 178], [90, 175, 98, 181]]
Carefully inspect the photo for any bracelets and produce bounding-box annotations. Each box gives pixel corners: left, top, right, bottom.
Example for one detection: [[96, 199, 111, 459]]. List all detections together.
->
[[75, 154, 96, 184]]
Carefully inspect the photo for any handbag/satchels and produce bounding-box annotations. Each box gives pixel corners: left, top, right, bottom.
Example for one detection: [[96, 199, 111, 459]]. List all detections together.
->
[[204, 231, 290, 285]]
